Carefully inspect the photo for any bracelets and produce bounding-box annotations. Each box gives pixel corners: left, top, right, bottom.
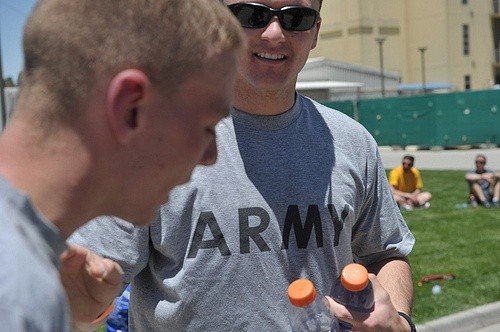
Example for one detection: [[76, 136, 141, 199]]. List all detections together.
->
[[90, 302, 114, 322], [492, 172, 495, 177], [480, 174, 483, 179], [398, 311, 417, 332]]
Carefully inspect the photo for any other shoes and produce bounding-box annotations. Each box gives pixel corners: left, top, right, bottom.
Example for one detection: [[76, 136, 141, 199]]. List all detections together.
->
[[402, 203, 413, 211], [492, 199, 500, 207], [421, 202, 430, 207], [482, 200, 491, 207]]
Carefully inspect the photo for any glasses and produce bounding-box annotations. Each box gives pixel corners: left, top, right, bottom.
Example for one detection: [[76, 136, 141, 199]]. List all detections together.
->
[[226, 2, 320, 32]]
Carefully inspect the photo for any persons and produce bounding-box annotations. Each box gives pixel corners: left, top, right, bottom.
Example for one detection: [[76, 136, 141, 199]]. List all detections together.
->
[[0, 0, 247, 332], [389, 156, 432, 211], [466, 155, 500, 208], [58, 0, 416, 332]]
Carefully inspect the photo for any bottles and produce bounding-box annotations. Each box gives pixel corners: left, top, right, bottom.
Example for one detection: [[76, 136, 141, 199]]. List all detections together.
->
[[287, 278, 340, 332], [331, 263, 375, 332]]
